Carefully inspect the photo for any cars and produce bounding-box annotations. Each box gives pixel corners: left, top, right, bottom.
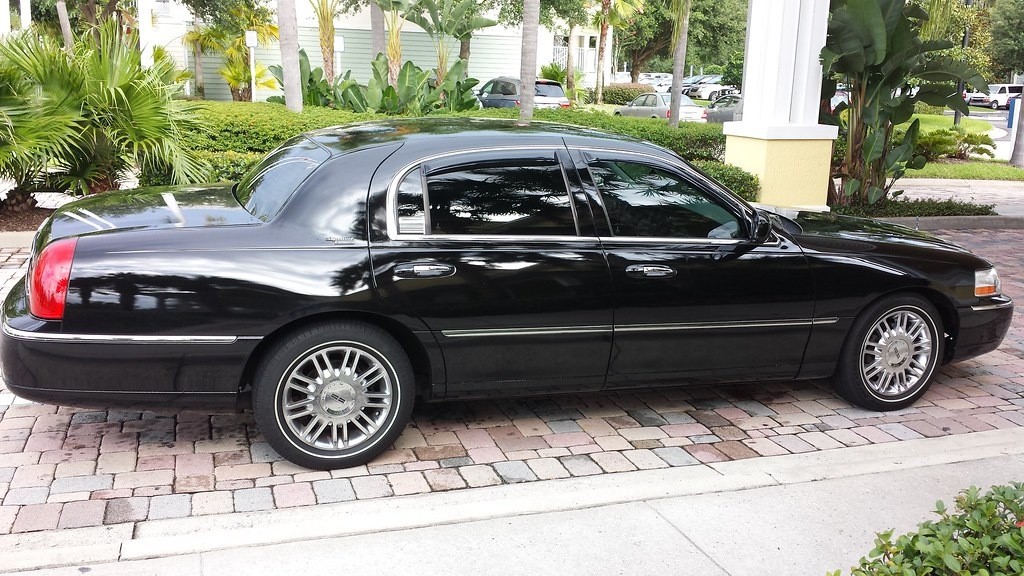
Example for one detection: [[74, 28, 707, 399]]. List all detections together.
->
[[705, 94, 743, 123], [637, 73, 740, 102], [614, 93, 707, 123], [0, 117, 1014, 472], [1005, 94, 1022, 110], [963, 89, 974, 106], [477, 76, 570, 109]]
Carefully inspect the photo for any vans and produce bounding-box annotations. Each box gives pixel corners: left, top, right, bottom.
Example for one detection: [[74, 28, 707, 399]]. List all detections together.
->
[[968, 84, 1023, 110]]
